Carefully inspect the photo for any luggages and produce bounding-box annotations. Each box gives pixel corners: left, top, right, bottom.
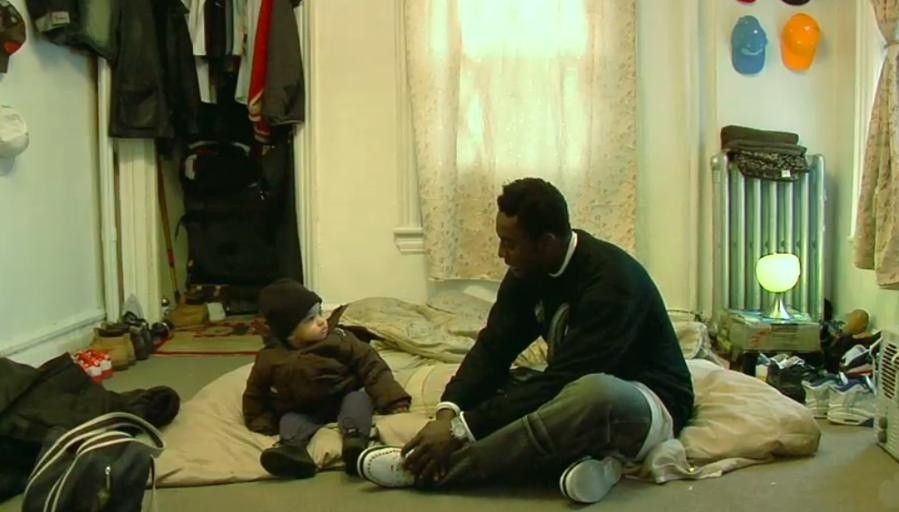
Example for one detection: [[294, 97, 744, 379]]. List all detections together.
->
[[183, 181, 280, 285]]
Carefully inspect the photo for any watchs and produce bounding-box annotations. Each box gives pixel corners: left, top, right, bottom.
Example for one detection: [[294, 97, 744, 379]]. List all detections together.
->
[[449, 416, 471, 444]]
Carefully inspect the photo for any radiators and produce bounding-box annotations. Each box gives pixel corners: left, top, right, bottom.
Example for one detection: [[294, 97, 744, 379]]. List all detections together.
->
[[710, 152, 825, 337]]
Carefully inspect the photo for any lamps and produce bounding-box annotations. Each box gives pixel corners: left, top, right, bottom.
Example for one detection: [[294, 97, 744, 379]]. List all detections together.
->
[[756, 253, 801, 319]]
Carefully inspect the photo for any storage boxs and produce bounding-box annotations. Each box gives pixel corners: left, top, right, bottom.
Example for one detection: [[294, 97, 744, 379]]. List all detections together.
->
[[728, 309, 822, 352]]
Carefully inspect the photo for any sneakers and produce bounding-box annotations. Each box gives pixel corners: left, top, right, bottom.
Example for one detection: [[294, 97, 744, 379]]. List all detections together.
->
[[556, 455, 623, 505], [260, 444, 316, 480], [71, 282, 228, 386], [357, 444, 417, 490], [342, 432, 369, 476], [741, 352, 875, 426]]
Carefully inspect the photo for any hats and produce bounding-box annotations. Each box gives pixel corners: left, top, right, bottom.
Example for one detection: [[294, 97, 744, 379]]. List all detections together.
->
[[0, 105, 30, 177], [732, 16, 765, 75], [258, 277, 322, 343], [0, 0, 27, 73], [781, 15, 821, 72]]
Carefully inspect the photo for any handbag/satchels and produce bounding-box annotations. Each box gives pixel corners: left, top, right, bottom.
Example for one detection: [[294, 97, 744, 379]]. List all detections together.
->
[[179, 136, 264, 193], [21, 409, 167, 512]]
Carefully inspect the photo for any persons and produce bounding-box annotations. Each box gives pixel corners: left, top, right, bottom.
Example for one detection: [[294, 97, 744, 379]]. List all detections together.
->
[[356, 179, 694, 502], [243, 279, 412, 475]]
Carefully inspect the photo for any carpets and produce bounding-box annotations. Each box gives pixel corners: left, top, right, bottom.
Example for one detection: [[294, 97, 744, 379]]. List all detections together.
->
[[149, 312, 269, 355]]
